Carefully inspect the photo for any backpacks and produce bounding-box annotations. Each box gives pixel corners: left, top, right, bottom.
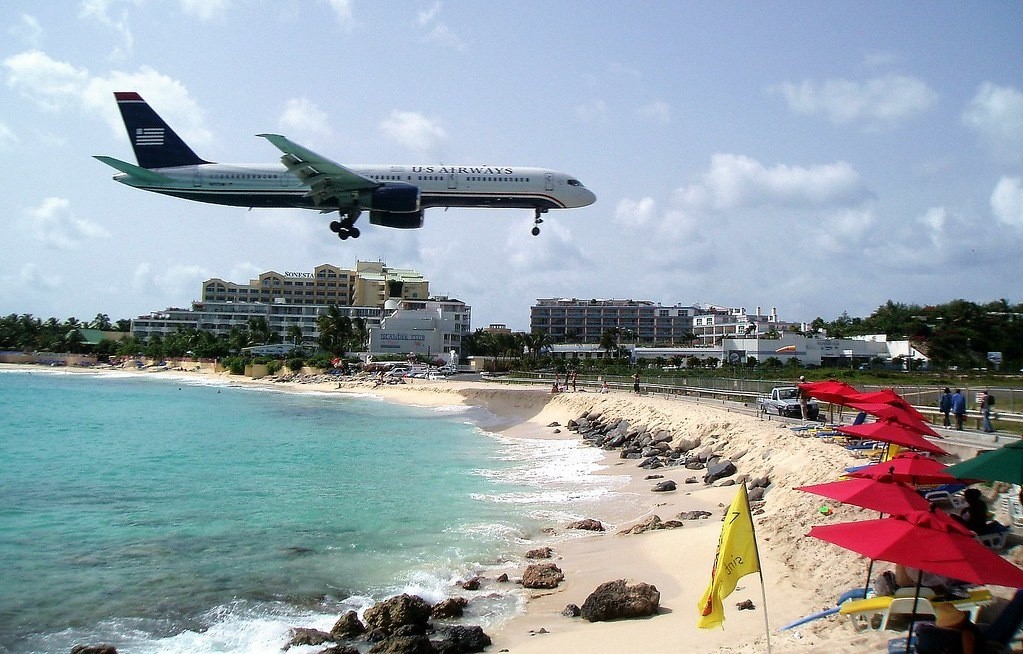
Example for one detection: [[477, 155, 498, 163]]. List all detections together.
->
[[987, 395, 995, 405]]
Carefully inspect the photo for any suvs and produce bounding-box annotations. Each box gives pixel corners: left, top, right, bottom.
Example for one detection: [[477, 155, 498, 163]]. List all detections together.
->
[[386, 368, 409, 377]]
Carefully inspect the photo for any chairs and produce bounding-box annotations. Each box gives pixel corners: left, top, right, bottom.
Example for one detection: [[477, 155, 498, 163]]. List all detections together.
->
[[840, 589, 997, 631], [888, 589, 1023, 654], [795, 422, 1013, 551]]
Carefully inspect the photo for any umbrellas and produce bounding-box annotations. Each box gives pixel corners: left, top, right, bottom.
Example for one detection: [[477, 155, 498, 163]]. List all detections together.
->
[[804, 502, 1023, 654], [938, 434, 1023, 487], [798, 377, 861, 424], [834, 416, 947, 464], [843, 387, 934, 424], [837, 451, 985, 487], [845, 395, 944, 440], [791, 465, 977, 602]]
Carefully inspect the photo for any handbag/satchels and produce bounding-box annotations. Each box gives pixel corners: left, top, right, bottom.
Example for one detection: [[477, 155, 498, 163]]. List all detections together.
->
[[962, 414, 968, 421]]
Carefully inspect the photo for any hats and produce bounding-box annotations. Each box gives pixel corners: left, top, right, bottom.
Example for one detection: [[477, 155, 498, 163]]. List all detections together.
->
[[800, 376, 805, 380], [935, 601, 964, 628]]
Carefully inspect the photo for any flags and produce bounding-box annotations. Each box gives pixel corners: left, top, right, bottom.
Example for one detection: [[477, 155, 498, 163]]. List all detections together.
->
[[696, 483, 759, 631]]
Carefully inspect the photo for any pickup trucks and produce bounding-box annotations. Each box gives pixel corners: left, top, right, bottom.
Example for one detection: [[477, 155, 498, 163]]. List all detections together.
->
[[757, 385, 819, 420]]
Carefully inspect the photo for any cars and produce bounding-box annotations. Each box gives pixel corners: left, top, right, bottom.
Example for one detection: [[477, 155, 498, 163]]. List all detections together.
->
[[404, 367, 437, 378], [425, 372, 446, 380]]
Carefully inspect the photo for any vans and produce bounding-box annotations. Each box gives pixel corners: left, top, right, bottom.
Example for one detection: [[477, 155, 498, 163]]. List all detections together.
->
[[437, 367, 451, 375]]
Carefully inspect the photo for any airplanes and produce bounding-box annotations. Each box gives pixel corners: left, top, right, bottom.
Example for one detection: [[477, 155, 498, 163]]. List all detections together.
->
[[91, 92, 596, 240]]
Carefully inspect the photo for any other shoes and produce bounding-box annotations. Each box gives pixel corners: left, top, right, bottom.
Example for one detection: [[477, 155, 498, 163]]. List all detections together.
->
[[942, 426, 947, 429], [801, 418, 807, 421], [947, 426, 951, 429]]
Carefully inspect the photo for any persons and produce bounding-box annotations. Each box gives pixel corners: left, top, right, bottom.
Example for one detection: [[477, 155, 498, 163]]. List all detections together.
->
[[631, 374, 641, 395], [931, 601, 1006, 654], [893, 563, 954, 593], [940, 387, 953, 429], [550, 371, 576, 393], [796, 376, 812, 421], [602, 381, 608, 394], [951, 389, 966, 431], [978, 389, 995, 433], [958, 489, 988, 528], [874, 563, 970, 596]]
